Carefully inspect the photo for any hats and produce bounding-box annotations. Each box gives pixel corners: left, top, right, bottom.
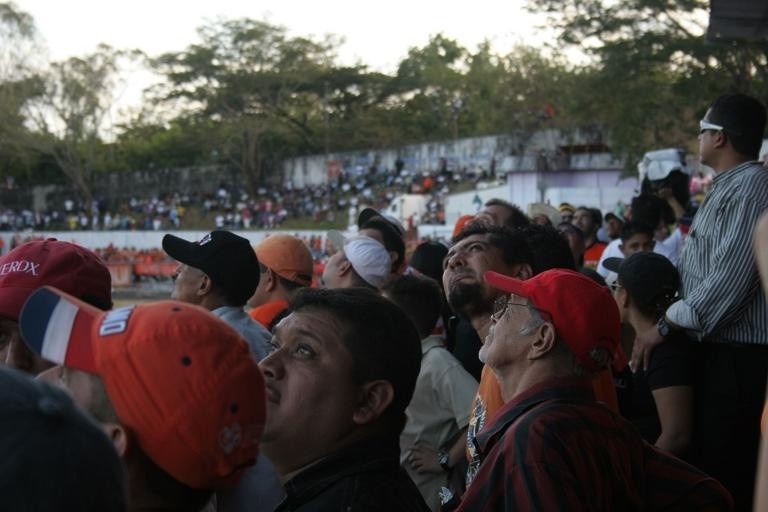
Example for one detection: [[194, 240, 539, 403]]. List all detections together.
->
[[254, 232, 313, 286], [483, 267, 623, 368], [526, 202, 563, 229], [0, 236, 113, 322], [357, 206, 406, 239], [327, 228, 392, 287], [161, 228, 262, 307], [601, 250, 682, 311]]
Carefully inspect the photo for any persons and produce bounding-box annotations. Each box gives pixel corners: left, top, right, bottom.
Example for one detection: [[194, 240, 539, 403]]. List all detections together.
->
[[244, 234, 313, 326], [625, 90, 767, 500], [137, 195, 190, 231], [516, 228, 574, 274], [467, 199, 531, 236], [388, 153, 499, 195], [163, 227, 274, 512], [258, 285, 431, 512], [421, 199, 446, 223], [603, 151, 716, 237], [203, 188, 289, 230], [601, 252, 722, 484], [320, 237, 391, 292], [443, 268, 736, 512], [0, 362, 126, 511], [603, 210, 625, 241], [281, 166, 395, 221], [0, 238, 113, 378], [529, 200, 589, 275], [605, 219, 657, 286], [0, 201, 61, 231], [411, 241, 450, 288], [356, 216, 406, 271], [64, 198, 138, 230], [381, 274, 483, 511], [442, 227, 534, 345], [573, 206, 610, 268]]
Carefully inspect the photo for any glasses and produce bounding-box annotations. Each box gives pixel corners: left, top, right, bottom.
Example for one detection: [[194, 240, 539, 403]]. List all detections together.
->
[[698, 119, 725, 134], [610, 280, 623, 291], [491, 300, 528, 318]]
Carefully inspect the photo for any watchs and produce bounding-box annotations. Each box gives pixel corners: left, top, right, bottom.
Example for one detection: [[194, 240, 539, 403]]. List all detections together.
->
[[658, 319, 671, 338], [437, 450, 452, 470]]
